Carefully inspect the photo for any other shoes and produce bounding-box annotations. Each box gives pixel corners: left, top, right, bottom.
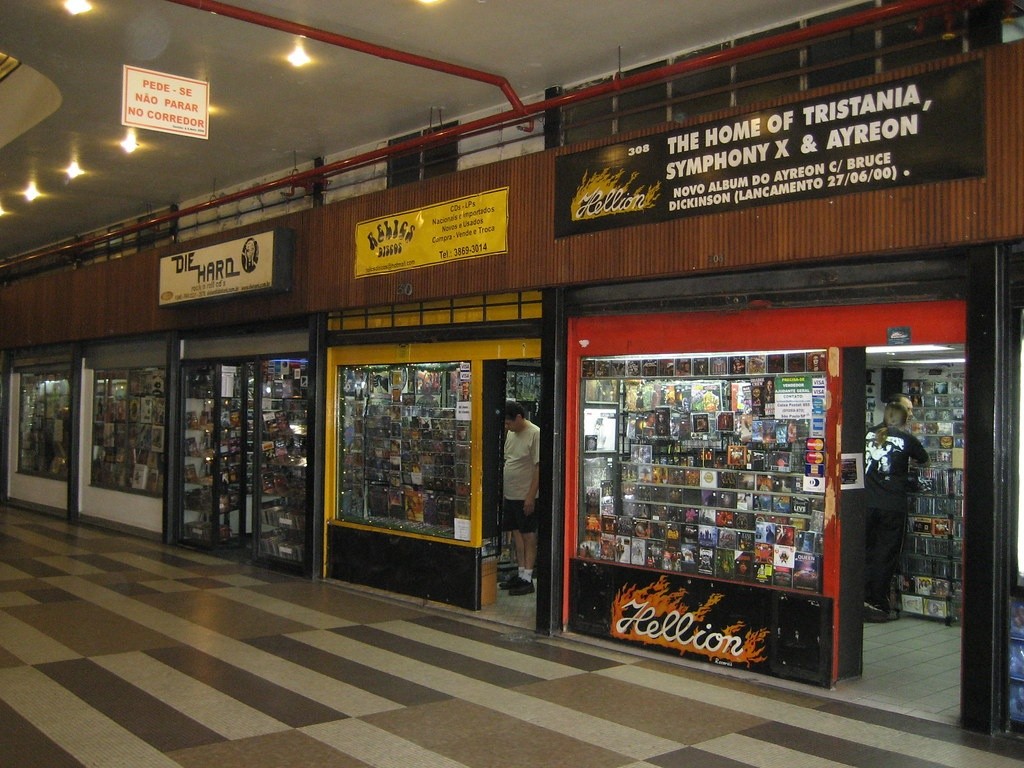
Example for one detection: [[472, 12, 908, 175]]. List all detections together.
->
[[864, 601, 898, 622]]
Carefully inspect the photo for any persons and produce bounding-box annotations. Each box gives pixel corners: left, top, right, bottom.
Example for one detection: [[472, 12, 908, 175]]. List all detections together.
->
[[764, 379, 776, 403], [862, 394, 932, 623], [498, 400, 540, 596]]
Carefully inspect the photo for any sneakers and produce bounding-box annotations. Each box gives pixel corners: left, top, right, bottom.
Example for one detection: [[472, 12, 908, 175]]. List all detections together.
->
[[499, 575, 534, 595]]
